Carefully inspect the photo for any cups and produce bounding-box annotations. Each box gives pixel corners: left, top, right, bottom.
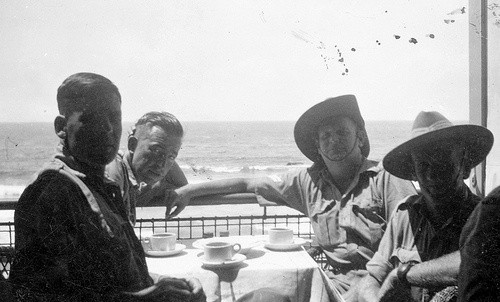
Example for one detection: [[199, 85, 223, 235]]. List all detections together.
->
[[143, 234, 176, 251], [203, 241, 241, 261], [269, 228, 293, 245]]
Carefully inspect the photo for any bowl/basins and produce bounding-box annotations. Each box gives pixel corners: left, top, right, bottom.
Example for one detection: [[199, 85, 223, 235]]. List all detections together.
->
[[191, 237, 260, 254]]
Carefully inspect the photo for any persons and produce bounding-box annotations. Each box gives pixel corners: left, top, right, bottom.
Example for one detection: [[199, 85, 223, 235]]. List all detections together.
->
[[16, 71, 207, 302], [358, 110, 500, 302], [104, 112, 189, 228], [164, 94, 418, 302]]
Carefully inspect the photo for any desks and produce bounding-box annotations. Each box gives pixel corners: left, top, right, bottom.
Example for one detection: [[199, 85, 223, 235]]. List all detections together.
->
[[141, 232, 345, 302]]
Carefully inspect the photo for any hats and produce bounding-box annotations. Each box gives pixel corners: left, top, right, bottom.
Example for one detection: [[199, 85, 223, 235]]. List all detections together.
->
[[383, 110, 494, 182], [294, 95, 370, 163]]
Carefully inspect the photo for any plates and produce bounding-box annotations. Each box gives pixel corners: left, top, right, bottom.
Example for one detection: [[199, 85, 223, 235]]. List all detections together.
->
[[196, 252, 247, 268], [142, 244, 186, 256], [257, 238, 306, 250]]
